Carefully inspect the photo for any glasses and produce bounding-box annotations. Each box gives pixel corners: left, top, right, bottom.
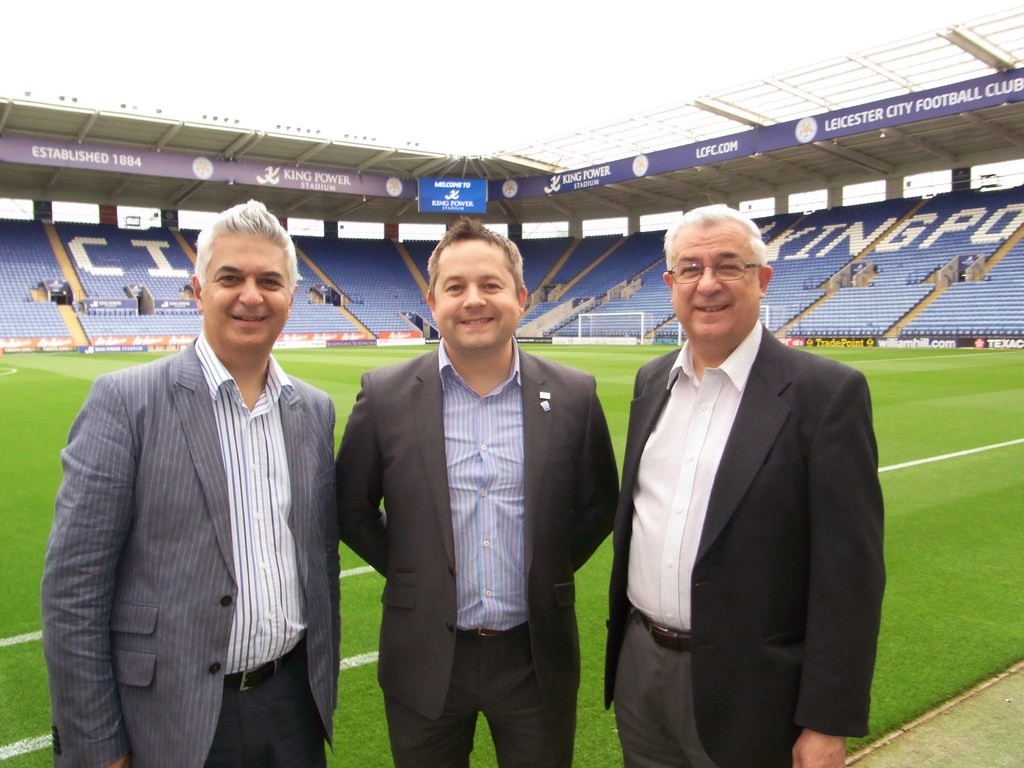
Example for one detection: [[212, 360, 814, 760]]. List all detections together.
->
[[668, 260, 761, 284]]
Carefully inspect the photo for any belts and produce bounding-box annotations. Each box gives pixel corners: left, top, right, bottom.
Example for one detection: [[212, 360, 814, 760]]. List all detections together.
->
[[637, 609, 691, 653], [224, 632, 307, 691], [456, 620, 527, 637]]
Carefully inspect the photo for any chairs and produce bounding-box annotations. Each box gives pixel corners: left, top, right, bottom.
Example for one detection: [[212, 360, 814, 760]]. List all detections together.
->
[[1, 181, 1024, 348]]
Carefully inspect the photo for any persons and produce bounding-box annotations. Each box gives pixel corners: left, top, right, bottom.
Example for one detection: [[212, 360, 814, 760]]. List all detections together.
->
[[42, 197, 340, 768], [603, 205, 888, 768], [337, 215, 621, 768]]
